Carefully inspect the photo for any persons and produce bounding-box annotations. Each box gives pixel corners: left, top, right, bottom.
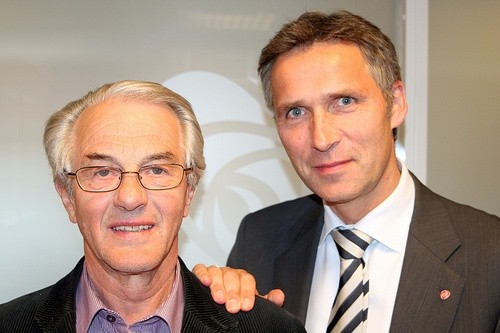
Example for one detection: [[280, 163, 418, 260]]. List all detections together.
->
[[192, 9, 500, 333], [0, 80, 306, 333]]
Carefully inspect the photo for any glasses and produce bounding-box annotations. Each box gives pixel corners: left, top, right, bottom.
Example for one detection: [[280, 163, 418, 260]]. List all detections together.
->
[[64, 164, 193, 192]]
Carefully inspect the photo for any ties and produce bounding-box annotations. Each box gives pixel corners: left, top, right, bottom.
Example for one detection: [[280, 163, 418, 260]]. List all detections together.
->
[[326, 228, 374, 333]]
